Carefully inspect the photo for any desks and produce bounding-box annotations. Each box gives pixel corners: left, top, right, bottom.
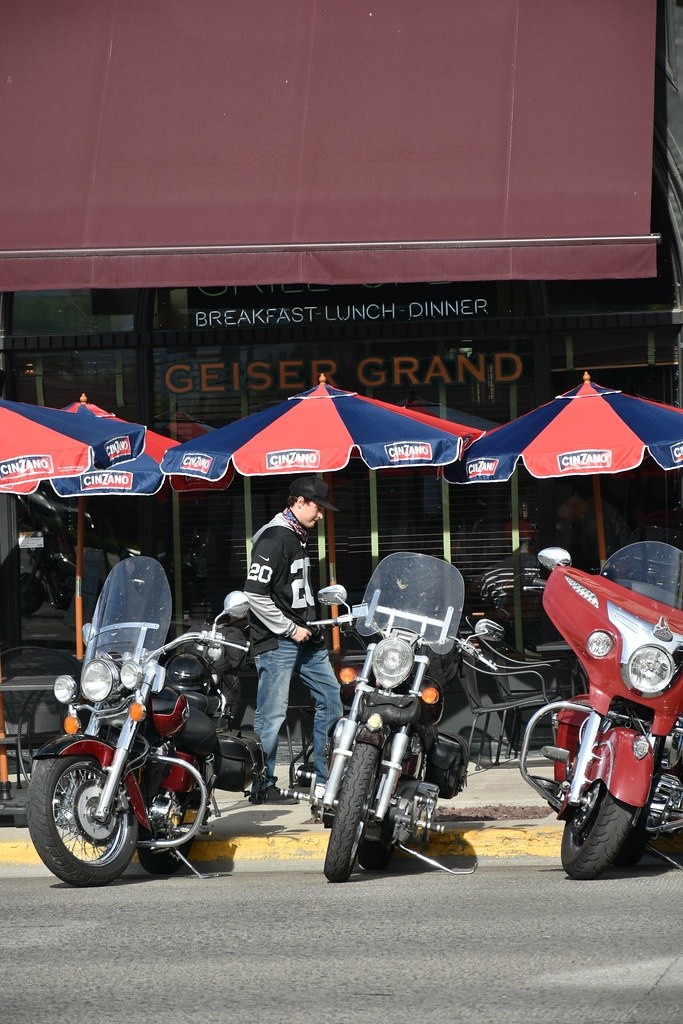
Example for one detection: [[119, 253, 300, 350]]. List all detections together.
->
[[535, 639, 572, 652], [0, 674, 76, 736]]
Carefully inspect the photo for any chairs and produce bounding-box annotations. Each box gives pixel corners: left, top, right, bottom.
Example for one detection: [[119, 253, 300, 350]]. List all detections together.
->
[[458, 657, 557, 773], [0, 645, 83, 789], [464, 614, 587, 760]]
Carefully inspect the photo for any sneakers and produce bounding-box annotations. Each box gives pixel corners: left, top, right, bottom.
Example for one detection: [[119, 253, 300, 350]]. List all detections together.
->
[[251, 785, 300, 806]]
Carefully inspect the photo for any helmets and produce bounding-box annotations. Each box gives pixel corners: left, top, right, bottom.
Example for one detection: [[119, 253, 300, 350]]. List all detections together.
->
[[164, 651, 212, 692]]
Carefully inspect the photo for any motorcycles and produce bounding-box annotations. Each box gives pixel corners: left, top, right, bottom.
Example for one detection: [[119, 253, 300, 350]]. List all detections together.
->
[[27, 556, 268, 888], [279, 552, 505, 883], [19, 530, 72, 616], [519, 542, 683, 880]]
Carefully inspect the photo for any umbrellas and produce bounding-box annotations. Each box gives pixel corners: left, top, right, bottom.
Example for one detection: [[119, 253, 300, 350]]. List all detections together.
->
[[437, 371, 683, 571], [149, 389, 497, 502], [159, 373, 483, 650], [0, 394, 181, 798]]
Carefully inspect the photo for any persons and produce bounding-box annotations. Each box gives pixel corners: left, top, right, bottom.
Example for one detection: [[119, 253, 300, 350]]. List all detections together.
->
[[241, 477, 346, 805], [553, 484, 629, 570]]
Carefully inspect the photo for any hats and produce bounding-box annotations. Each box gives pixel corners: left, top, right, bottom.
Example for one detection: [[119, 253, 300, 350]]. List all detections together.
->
[[504, 520, 534, 541], [290, 474, 340, 513], [554, 486, 579, 505]]
